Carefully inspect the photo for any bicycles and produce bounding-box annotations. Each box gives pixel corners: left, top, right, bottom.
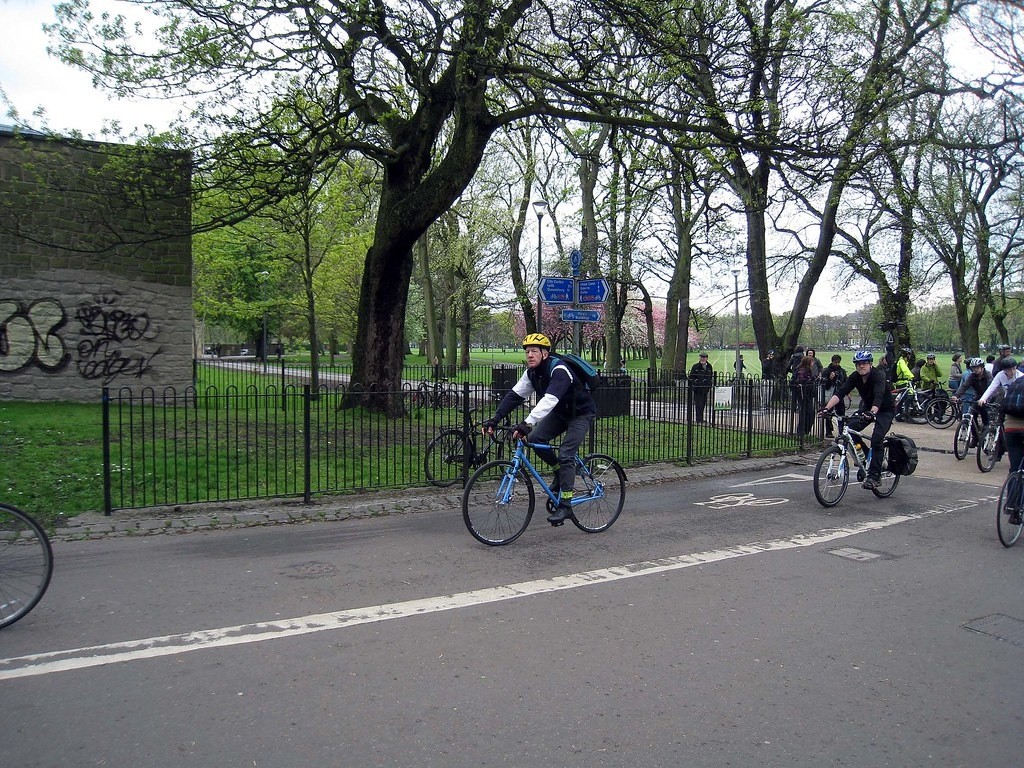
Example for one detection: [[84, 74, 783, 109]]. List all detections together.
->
[[0, 502, 54, 630], [403, 375, 459, 409], [813, 412, 900, 508], [424, 393, 529, 488], [462, 422, 629, 546], [890, 380, 1024, 548], [780, 384, 852, 411]]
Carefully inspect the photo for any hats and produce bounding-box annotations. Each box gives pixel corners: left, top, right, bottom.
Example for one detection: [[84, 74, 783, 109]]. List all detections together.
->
[[699, 352, 708, 357], [767, 350, 774, 355]]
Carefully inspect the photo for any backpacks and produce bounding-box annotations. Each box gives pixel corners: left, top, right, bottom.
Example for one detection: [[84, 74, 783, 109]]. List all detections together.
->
[[1004, 375, 1024, 418], [549, 353, 603, 393], [886, 432, 918, 476], [932, 388, 950, 418], [890, 355, 903, 382]]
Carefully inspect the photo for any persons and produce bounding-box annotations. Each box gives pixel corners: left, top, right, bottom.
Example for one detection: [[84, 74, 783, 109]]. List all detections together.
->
[[276, 344, 282, 360], [783, 346, 849, 439], [761, 349, 777, 410], [210, 342, 222, 362], [603, 352, 627, 374], [875, 347, 944, 424], [949, 344, 1024, 525], [817, 351, 896, 490], [688, 351, 713, 426], [732, 355, 747, 381], [481, 332, 598, 523]]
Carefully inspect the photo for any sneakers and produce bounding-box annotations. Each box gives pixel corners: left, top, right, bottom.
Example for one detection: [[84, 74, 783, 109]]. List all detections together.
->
[[546, 467, 572, 522], [854, 461, 882, 489]]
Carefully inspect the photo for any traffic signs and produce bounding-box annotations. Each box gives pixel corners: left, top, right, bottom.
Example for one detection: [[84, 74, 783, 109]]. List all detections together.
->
[[536, 277, 610, 322]]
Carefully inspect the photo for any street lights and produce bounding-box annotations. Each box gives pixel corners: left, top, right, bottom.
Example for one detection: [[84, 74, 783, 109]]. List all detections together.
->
[[532, 199, 548, 333], [731, 270, 742, 378], [261, 271, 269, 372]]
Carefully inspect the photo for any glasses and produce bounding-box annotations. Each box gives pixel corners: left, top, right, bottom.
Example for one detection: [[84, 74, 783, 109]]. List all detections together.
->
[[769, 354, 774, 356]]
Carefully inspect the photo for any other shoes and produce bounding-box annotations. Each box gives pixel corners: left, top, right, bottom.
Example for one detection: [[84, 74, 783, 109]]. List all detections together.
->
[[826, 432, 835, 438], [969, 437, 1001, 462]]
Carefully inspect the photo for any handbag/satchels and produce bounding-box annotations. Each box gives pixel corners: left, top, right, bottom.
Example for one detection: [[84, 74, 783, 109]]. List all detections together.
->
[[824, 385, 836, 404]]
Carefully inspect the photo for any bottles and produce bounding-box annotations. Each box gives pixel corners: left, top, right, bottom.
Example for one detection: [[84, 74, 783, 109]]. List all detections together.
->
[[977, 415, 983, 435], [865, 447, 872, 470], [855, 444, 866, 461]]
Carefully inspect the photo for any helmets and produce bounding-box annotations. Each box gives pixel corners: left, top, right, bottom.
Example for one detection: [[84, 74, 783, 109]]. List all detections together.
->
[[522, 333, 552, 349], [901, 347, 913, 355], [999, 344, 1010, 356], [852, 351, 873, 362], [927, 353, 936, 358], [963, 357, 984, 368], [1001, 357, 1018, 369]]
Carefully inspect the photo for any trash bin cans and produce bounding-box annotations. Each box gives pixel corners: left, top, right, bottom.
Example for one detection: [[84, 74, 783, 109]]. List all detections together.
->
[[492, 368, 517, 410], [596, 375, 631, 418]]
